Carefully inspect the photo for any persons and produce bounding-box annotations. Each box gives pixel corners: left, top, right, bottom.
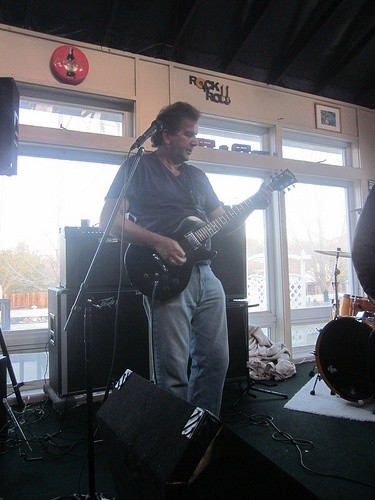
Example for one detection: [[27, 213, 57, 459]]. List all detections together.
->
[[100, 102, 272, 418]]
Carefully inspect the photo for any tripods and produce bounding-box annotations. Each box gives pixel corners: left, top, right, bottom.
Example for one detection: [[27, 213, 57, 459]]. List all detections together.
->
[[227, 303, 289, 409]]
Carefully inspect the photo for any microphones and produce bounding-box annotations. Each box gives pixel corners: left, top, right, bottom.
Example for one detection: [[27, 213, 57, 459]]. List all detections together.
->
[[129, 118, 164, 151]]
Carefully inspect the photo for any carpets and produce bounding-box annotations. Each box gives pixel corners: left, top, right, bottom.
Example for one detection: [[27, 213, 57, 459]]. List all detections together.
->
[[283, 373, 375, 422]]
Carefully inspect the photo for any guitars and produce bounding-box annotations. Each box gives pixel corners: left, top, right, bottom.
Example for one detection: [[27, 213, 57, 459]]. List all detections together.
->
[[123, 168, 298, 302]]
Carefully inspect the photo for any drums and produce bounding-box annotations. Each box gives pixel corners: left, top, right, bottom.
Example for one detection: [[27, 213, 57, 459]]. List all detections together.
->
[[313, 314, 375, 403], [339, 294, 375, 320]]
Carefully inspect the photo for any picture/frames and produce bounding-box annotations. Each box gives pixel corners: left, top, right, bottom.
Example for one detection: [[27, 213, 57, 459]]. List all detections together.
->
[[314, 103, 342, 133]]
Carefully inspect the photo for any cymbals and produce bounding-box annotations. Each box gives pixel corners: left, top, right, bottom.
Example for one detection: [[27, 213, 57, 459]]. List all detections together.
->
[[312, 250, 351, 257]]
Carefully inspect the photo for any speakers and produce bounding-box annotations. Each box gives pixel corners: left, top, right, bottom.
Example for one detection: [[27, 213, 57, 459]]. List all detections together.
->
[[0, 77, 19, 176], [46, 212, 318, 500]]
